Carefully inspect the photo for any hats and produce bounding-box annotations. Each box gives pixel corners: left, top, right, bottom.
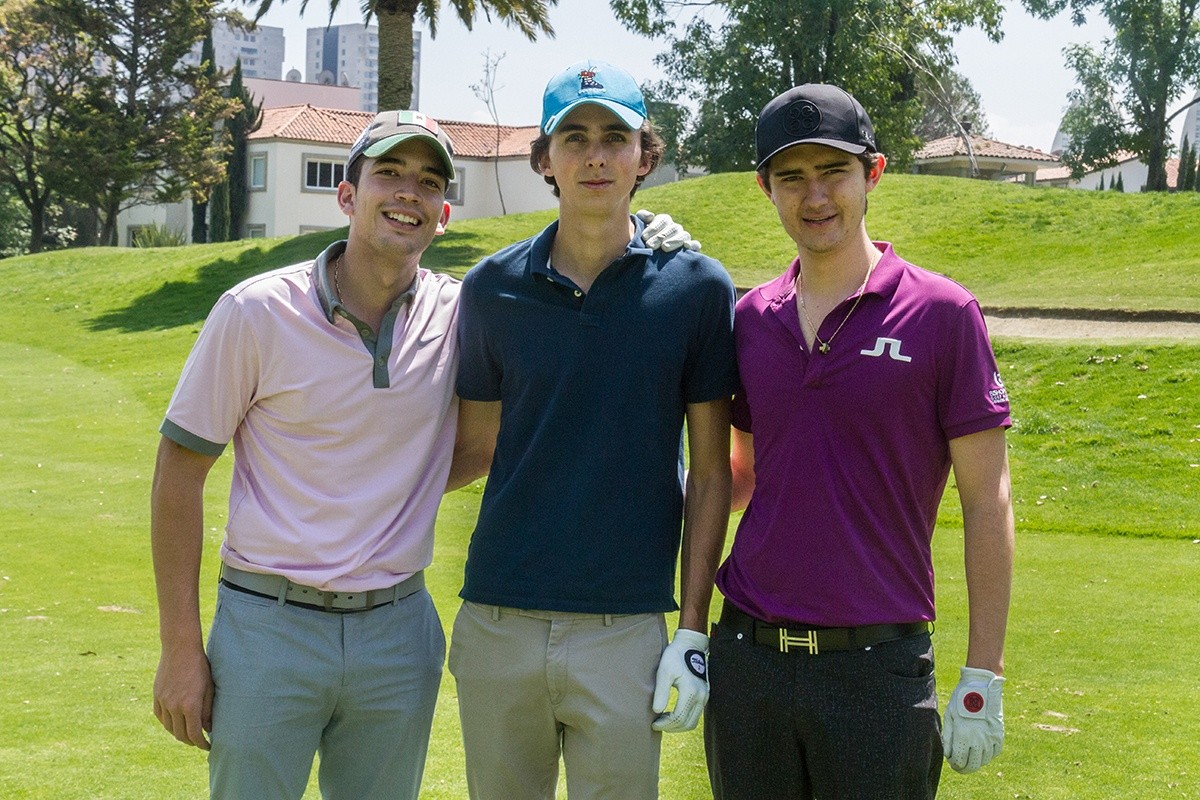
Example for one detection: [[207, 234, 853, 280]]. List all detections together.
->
[[540, 56, 648, 135], [344, 109, 455, 180], [755, 83, 876, 172]]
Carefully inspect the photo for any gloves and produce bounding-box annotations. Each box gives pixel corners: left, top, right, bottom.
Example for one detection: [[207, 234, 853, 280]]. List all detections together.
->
[[652, 628, 709, 732], [942, 667, 1007, 775], [637, 209, 701, 253]]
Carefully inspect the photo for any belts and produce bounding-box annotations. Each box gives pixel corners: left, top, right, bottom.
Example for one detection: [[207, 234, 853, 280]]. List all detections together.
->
[[719, 598, 928, 654], [221, 563, 425, 611]]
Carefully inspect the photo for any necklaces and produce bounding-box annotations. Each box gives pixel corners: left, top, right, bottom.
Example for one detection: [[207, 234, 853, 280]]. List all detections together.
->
[[798, 247, 877, 353], [334, 252, 343, 305]]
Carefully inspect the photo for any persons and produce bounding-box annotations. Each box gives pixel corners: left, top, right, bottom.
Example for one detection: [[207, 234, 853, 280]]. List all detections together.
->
[[151, 109, 704, 800], [444, 62, 744, 800], [692, 81, 1017, 800]]
